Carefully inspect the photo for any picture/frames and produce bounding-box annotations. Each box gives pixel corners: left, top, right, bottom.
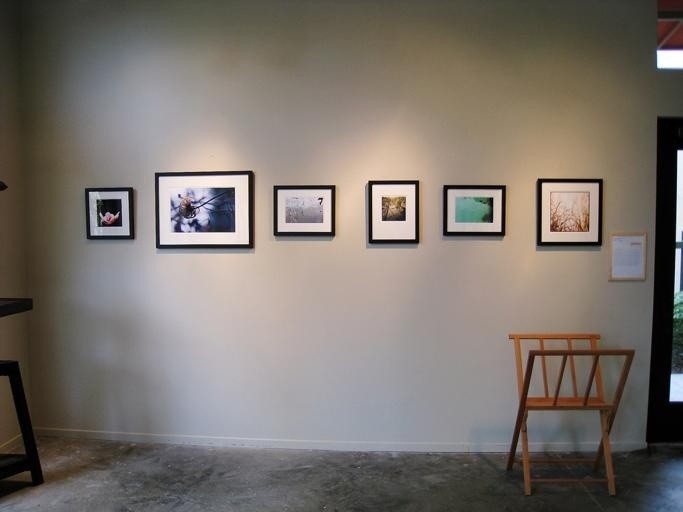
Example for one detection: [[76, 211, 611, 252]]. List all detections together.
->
[[536, 178, 604, 247], [368, 180, 420, 244], [442, 184, 506, 237], [154, 170, 254, 249], [607, 231, 649, 282], [272, 184, 336, 237], [84, 186, 135, 240]]
